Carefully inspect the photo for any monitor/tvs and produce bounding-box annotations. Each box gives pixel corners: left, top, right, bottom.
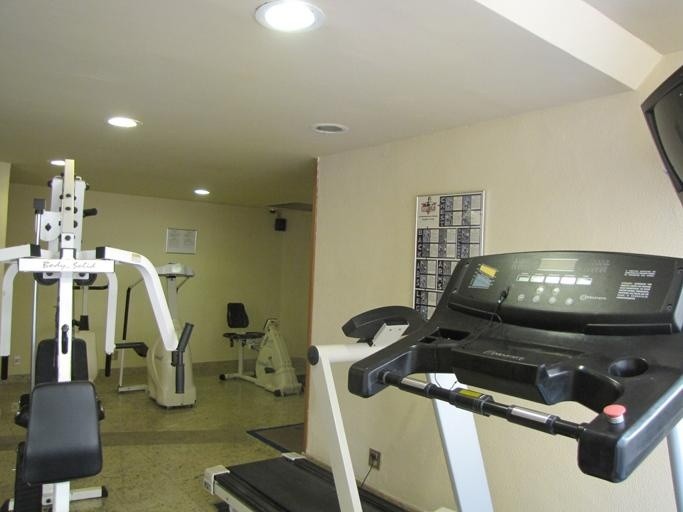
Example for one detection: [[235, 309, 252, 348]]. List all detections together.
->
[[640, 65, 683, 206]]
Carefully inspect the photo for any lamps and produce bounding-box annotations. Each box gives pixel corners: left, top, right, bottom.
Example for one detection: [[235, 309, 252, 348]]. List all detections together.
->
[[271, 209, 287, 231], [254, 1, 325, 34]]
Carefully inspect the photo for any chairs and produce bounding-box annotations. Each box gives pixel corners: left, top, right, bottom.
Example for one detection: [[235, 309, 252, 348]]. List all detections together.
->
[[21, 336, 108, 512], [219, 303, 266, 380]]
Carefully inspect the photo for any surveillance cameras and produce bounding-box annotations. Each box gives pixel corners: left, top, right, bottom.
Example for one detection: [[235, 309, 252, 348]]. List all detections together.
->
[[269, 208, 276, 213]]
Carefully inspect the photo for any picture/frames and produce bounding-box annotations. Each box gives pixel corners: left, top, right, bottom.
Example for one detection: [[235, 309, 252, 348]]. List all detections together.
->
[[165, 226, 197, 255]]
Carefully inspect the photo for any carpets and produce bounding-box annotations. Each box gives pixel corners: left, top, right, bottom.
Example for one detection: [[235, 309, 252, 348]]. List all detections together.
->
[[246, 422, 305, 454]]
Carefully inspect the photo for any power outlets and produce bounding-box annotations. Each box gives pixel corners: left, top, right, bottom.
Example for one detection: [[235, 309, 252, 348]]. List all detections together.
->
[[368, 448, 381, 470]]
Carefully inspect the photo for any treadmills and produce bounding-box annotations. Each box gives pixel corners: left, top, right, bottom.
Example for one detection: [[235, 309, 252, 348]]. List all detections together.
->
[[204, 305, 493, 512]]
